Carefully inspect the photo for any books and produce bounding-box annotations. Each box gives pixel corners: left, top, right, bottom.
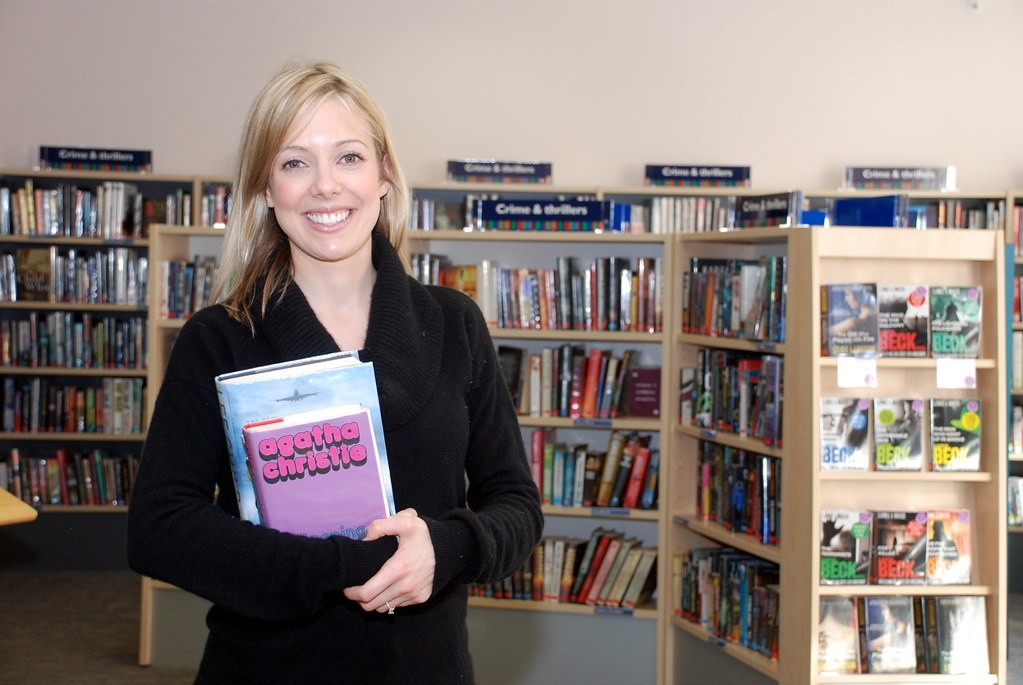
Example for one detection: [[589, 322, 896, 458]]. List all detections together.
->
[[409, 197, 1023, 685], [0, 182, 241, 507], [215, 350, 396, 540]]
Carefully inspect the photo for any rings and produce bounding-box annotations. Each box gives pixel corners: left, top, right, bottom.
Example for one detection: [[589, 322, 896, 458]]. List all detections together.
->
[[386, 602, 395, 614]]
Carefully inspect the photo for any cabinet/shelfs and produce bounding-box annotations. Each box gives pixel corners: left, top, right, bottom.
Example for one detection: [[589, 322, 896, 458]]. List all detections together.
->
[[0, 167, 1023, 685]]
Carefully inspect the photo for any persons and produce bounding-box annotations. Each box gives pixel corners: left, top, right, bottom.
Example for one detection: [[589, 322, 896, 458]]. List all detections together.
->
[[131, 63, 545, 685]]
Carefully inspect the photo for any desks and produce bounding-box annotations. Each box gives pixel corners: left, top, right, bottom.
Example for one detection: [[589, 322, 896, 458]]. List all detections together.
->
[[0, 486, 37, 525]]
